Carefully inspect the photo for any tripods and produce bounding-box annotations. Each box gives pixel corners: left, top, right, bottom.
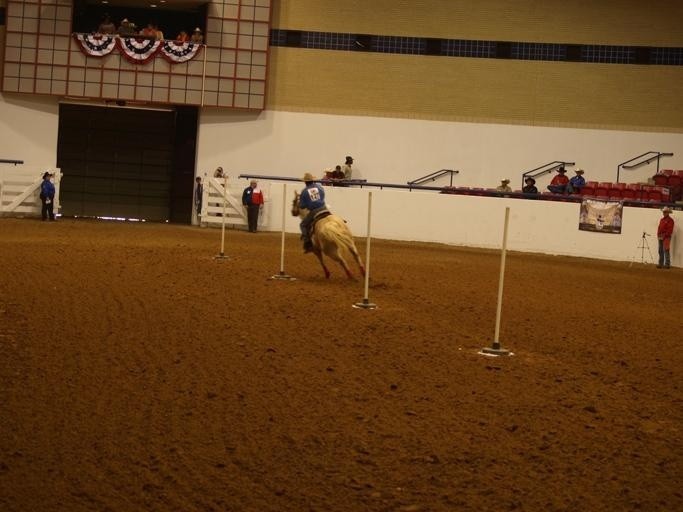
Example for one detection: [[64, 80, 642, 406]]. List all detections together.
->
[[631, 236, 655, 263]]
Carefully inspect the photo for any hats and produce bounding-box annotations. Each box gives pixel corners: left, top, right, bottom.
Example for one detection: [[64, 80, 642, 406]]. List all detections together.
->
[[556, 166, 566, 173], [525, 176, 535, 184], [501, 178, 510, 184], [575, 167, 584, 174], [345, 155, 354, 164], [195, 28, 201, 33], [43, 172, 52, 179], [661, 206, 672, 213], [302, 173, 317, 182], [121, 19, 136, 27]]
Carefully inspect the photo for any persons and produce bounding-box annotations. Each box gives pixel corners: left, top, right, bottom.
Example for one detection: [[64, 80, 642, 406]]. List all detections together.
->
[[214, 167, 229, 216], [496, 178, 512, 192], [40, 172, 55, 222], [300, 173, 329, 253], [565, 168, 585, 194], [523, 178, 538, 194], [547, 167, 570, 194], [656, 206, 674, 269], [194, 177, 203, 214], [242, 179, 264, 233], [321, 156, 354, 187]]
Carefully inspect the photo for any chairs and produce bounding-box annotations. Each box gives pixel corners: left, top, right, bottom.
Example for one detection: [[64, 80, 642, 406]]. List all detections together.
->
[[439, 169, 683, 210]]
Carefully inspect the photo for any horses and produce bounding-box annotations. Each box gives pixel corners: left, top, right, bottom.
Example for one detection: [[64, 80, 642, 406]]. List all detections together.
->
[[291, 189, 366, 280]]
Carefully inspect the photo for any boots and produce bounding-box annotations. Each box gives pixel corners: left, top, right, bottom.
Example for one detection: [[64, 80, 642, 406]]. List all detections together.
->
[[303, 242, 314, 254]]
